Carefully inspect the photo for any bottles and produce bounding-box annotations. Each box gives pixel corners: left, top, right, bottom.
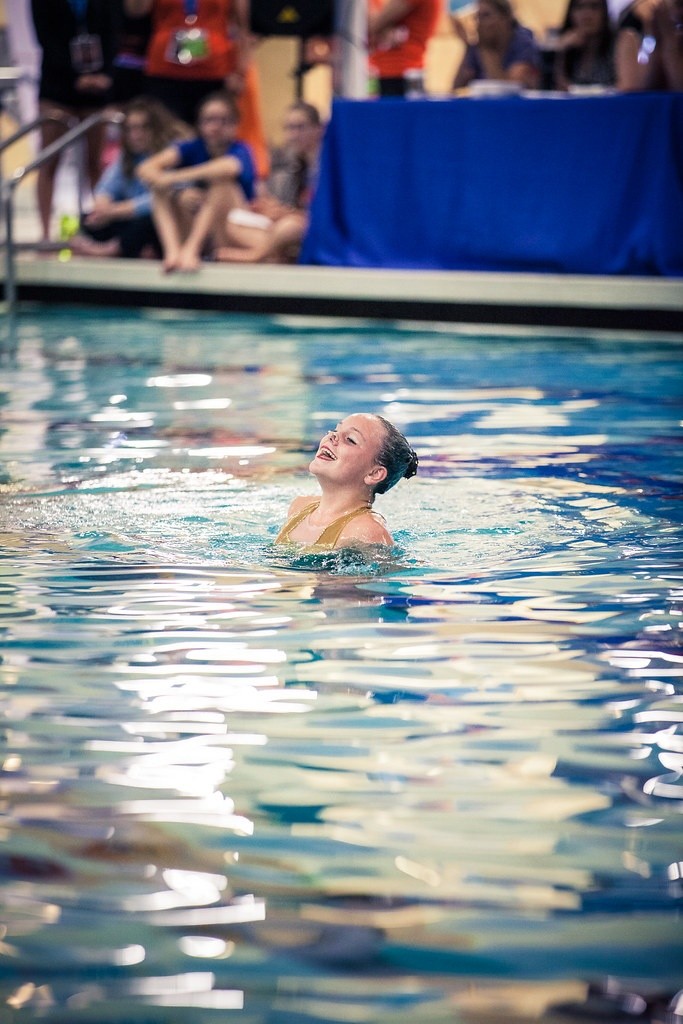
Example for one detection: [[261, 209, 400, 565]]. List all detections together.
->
[[55, 215, 74, 260]]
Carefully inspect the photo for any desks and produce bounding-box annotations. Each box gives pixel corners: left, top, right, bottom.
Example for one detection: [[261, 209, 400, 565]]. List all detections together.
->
[[297, 90, 683, 282]]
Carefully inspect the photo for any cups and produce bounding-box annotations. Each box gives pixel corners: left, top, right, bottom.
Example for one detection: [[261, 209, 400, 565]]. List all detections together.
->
[[405, 69, 425, 101]]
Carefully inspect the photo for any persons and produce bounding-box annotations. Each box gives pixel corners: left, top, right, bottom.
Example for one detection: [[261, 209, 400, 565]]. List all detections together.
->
[[8, 0, 682, 280], [273, 411, 422, 544]]
